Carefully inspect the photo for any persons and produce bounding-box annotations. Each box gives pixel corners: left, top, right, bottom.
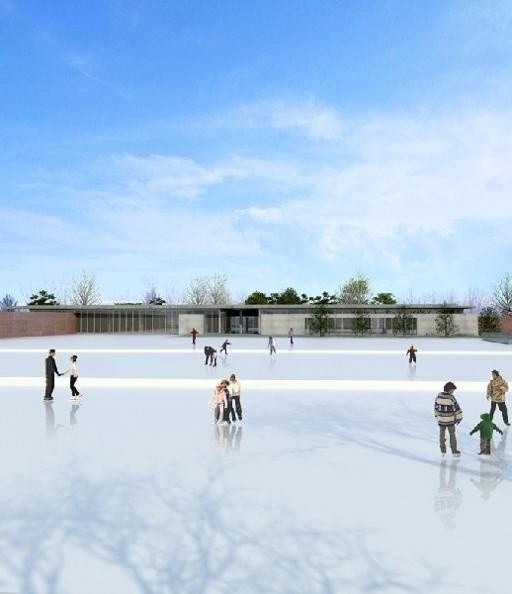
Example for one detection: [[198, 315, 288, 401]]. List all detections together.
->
[[486, 369, 511, 426], [470, 462, 512, 500], [434, 381, 463, 456], [210, 374, 242, 425], [190, 328, 199, 343], [289, 328, 294, 344], [267, 335, 276, 354], [469, 413, 504, 455], [406, 345, 418, 366], [204, 341, 231, 366], [44, 349, 61, 399], [434, 459, 461, 529], [63, 355, 81, 398]]
